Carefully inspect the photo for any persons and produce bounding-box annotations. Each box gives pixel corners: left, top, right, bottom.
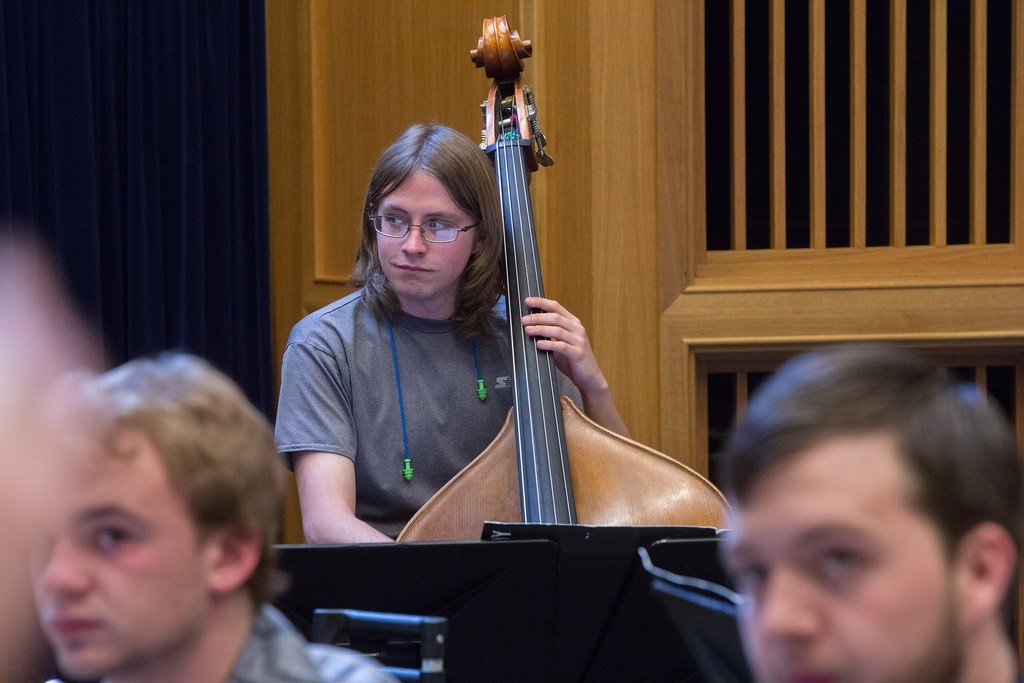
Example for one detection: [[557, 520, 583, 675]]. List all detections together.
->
[[272, 124, 632, 545], [32, 357, 396, 682], [721, 345, 1024, 683]]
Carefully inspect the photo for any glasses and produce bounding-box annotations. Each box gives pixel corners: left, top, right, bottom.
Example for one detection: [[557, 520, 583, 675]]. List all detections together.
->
[[369, 207, 482, 243]]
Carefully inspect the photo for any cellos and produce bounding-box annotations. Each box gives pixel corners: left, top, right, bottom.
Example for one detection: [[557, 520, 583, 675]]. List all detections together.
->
[[386, 13, 736, 532]]
